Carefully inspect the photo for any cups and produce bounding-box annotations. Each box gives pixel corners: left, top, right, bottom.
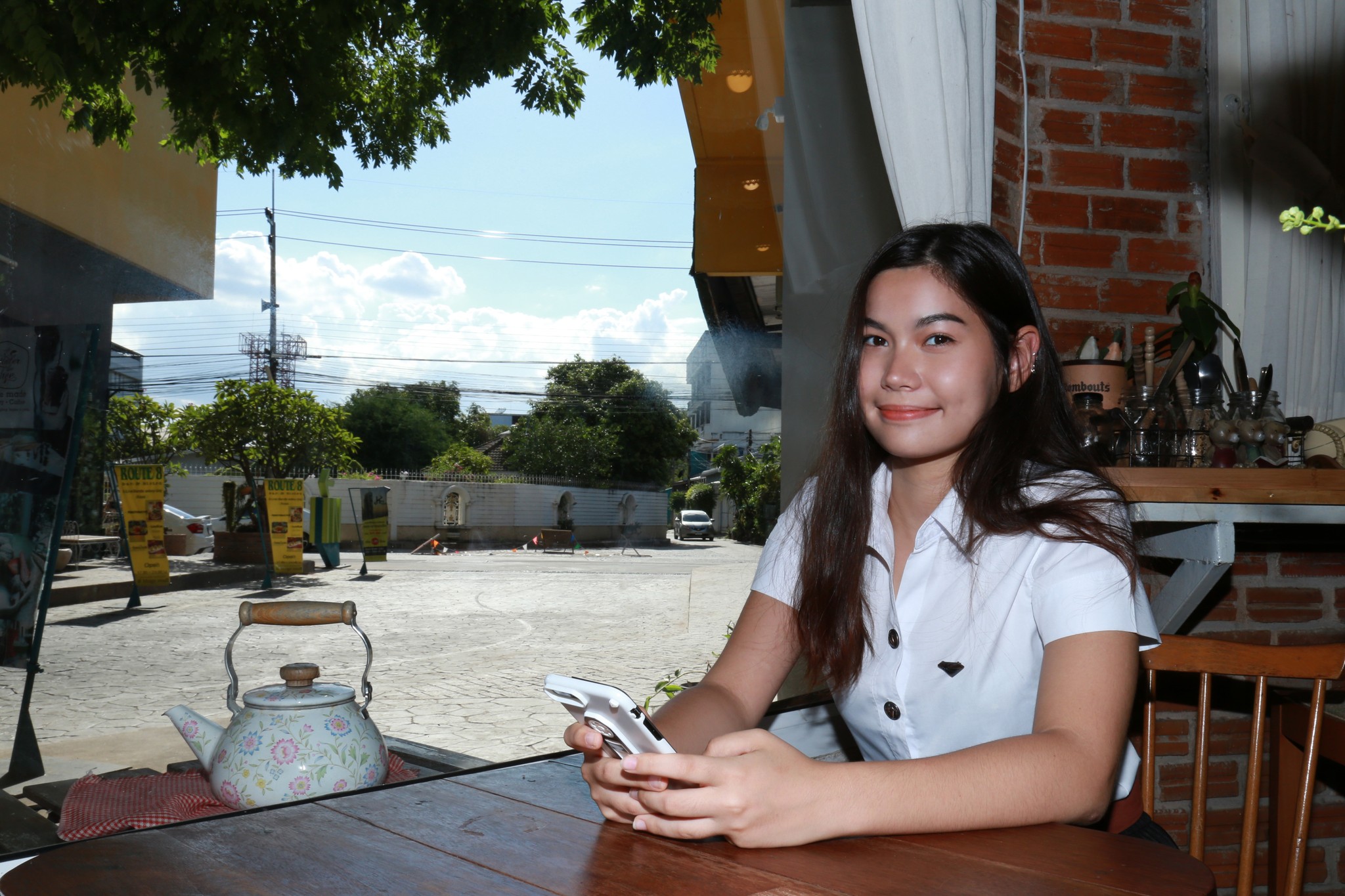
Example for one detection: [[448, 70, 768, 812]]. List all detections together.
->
[[1056, 360, 1130, 466]]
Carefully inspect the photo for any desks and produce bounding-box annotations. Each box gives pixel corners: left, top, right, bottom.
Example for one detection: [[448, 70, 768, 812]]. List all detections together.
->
[[0, 746, 1219, 896], [62, 534, 122, 564], [1270, 695, 1345, 896]]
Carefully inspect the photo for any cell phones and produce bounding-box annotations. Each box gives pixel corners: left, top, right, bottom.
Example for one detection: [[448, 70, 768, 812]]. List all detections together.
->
[[543, 674, 698, 787]]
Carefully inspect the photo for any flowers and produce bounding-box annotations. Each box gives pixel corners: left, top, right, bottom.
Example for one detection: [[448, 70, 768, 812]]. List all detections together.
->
[[1140, 269, 1243, 356]]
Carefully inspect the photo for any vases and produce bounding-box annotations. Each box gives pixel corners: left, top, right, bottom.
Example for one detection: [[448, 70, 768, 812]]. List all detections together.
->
[[1175, 353, 1223, 389], [56, 548, 72, 570]]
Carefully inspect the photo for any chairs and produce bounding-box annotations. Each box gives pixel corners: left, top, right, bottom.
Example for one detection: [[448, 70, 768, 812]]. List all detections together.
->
[[1140, 634, 1345, 896], [103, 514, 122, 559], [60, 521, 79, 572]]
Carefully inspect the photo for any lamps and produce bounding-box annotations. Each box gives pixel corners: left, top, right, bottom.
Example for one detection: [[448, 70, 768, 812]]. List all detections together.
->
[[755, 94, 785, 130]]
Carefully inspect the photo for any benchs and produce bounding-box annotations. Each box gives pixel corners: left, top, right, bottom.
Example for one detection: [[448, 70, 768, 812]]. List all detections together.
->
[[0, 732, 498, 863]]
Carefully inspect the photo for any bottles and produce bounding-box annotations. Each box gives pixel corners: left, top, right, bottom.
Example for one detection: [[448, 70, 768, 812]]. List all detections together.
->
[[1066, 385, 1287, 467]]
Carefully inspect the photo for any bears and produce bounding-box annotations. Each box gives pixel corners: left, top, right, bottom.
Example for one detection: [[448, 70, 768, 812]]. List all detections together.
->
[[1208, 418, 1290, 469]]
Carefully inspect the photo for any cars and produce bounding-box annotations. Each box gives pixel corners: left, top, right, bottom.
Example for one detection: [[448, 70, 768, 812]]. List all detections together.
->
[[674, 510, 715, 541], [103, 501, 216, 557]]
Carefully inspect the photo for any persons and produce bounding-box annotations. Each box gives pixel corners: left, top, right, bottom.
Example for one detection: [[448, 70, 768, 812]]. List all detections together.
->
[[563, 219, 1180, 850]]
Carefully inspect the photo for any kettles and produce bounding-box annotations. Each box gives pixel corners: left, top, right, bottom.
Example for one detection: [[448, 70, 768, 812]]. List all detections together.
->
[[161, 600, 391, 812]]
